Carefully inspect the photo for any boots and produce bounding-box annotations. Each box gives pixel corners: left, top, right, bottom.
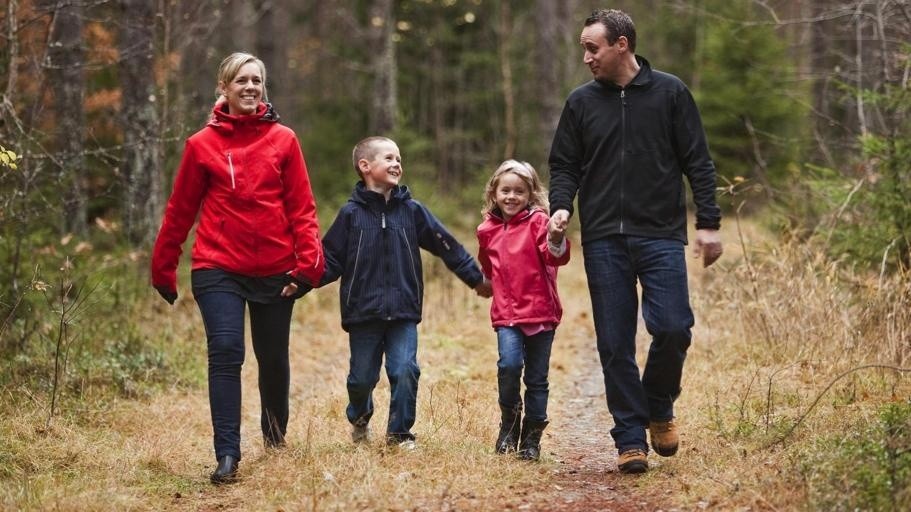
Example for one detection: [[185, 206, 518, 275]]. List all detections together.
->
[[494, 404, 548, 461]]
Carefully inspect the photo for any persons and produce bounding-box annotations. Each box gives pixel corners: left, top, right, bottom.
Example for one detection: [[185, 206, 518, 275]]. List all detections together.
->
[[547, 8, 723, 476], [473, 156, 572, 462], [280, 135, 493, 455], [153, 50, 325, 488]]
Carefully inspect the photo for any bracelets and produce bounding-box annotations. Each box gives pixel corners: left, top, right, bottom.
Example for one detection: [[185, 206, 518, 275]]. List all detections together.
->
[[552, 241, 562, 244]]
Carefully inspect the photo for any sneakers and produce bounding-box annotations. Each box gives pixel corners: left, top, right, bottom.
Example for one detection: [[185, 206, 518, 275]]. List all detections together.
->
[[618, 449, 650, 475], [649, 419, 680, 457]]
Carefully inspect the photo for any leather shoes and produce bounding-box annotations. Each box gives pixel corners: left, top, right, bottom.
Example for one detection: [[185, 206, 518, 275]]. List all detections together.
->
[[209, 456, 238, 483]]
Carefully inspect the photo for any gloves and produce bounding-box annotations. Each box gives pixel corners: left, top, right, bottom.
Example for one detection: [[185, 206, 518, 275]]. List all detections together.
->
[[270, 273, 311, 299], [154, 286, 179, 306]]
